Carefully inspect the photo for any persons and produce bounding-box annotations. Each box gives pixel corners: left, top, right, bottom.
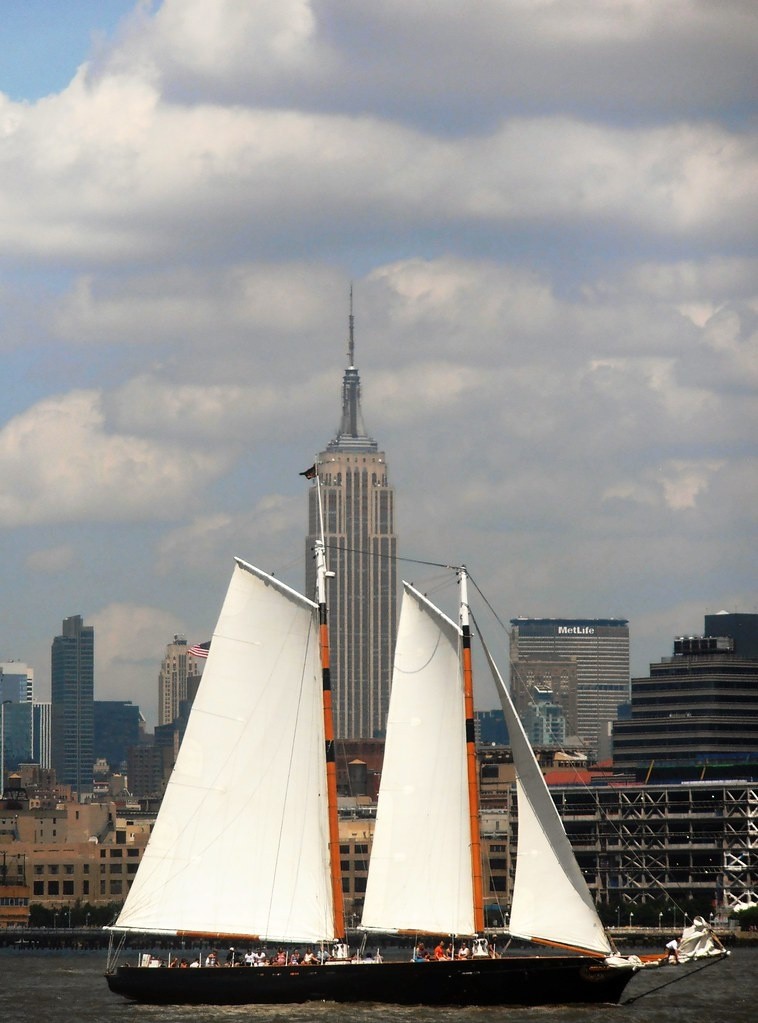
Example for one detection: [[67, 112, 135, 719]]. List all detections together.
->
[[666, 938, 681, 964], [408, 934, 500, 962], [152, 946, 332, 967]]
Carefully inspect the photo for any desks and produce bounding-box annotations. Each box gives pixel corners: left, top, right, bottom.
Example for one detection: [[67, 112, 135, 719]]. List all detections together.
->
[[325, 957, 352, 963]]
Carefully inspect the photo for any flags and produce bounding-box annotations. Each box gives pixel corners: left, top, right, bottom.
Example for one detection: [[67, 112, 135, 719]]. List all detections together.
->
[[187, 641, 211, 659], [299, 464, 318, 480]]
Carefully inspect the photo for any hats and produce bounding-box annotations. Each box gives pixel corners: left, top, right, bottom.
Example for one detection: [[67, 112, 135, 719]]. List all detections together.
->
[[492, 934, 497, 939], [229, 948, 235, 951]]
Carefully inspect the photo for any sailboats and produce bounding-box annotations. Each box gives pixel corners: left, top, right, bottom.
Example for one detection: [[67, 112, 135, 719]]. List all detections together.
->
[[101, 451, 734, 1005]]
